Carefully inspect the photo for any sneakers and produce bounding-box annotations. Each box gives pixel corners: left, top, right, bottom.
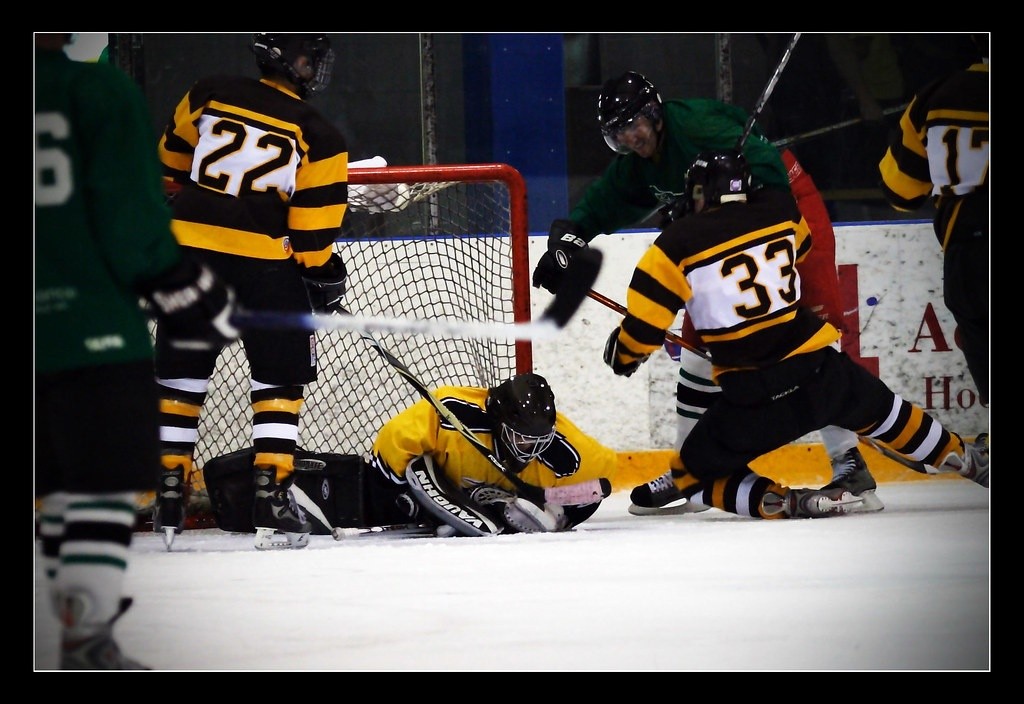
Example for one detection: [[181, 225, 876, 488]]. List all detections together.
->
[[762, 488, 864, 518], [153, 464, 185, 552], [628, 471, 711, 515], [252, 465, 313, 550], [53, 587, 152, 670], [938, 433, 989, 488], [819, 447, 884, 512]]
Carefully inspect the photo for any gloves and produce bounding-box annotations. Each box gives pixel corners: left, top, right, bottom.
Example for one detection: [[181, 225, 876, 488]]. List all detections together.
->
[[305, 254, 347, 315], [603, 328, 650, 377], [534, 218, 588, 294], [139, 252, 265, 342]]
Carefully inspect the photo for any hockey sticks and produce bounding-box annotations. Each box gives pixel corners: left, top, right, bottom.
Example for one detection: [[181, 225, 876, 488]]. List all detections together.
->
[[637, 33, 911, 224], [332, 519, 435, 541], [586, 287, 939, 474], [333, 303, 612, 506], [142, 247, 602, 341]]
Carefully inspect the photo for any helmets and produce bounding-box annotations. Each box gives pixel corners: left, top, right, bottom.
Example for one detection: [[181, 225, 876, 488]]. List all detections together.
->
[[486, 374, 556, 473], [685, 149, 753, 214], [251, 32, 335, 97], [598, 71, 664, 135]]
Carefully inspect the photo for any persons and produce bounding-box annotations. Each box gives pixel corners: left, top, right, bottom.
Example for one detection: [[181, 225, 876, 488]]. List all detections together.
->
[[203, 373, 674, 536], [34, 33, 352, 670], [532, 36, 989, 519]]
